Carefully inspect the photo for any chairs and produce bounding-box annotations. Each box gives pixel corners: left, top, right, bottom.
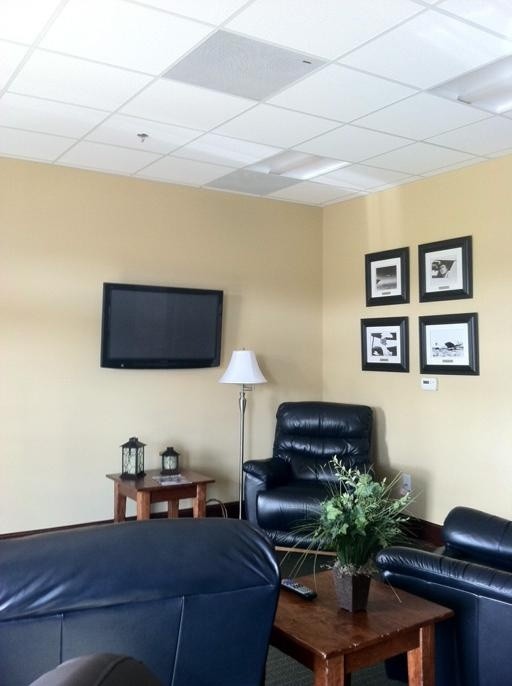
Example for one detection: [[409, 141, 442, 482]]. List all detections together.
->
[[240, 400, 375, 558], [374, 504, 512, 685]]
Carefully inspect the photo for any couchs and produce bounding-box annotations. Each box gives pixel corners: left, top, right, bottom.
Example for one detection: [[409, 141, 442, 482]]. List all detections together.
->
[[0, 516, 283, 685]]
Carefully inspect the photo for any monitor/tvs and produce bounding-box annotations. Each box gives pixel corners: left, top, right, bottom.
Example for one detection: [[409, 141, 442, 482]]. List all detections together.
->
[[99, 281, 224, 371]]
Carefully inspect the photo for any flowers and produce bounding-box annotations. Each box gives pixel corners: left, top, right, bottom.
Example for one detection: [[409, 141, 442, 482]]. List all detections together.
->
[[272, 447, 425, 580]]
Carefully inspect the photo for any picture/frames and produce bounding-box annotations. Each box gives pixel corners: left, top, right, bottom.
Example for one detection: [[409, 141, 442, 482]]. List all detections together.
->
[[360, 234, 480, 375]]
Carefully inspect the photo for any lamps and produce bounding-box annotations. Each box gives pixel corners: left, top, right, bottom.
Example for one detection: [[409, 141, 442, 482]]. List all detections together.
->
[[215, 346, 268, 524]]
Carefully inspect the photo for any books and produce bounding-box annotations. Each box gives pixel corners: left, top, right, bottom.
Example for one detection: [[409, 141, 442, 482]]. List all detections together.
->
[[151, 475, 193, 488]]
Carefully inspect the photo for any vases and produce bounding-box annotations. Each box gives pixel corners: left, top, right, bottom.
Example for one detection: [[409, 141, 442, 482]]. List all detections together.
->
[[333, 566, 371, 612]]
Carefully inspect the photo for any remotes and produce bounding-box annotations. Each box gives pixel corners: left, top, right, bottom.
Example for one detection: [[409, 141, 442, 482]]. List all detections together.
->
[[281, 576, 318, 602]]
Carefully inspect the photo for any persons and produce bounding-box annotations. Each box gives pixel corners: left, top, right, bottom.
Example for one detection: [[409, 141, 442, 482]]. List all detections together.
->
[[437, 263, 450, 277]]
